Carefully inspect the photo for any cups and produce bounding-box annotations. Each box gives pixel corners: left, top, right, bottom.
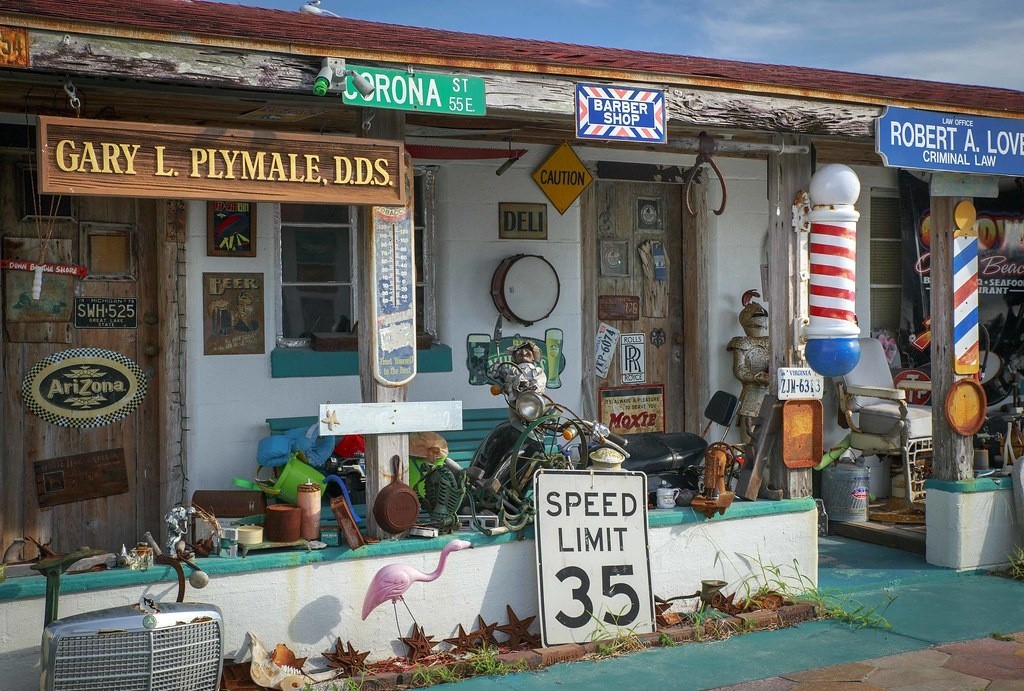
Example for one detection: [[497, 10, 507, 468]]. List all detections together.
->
[[467, 333, 490, 385], [544, 327, 564, 388], [657, 488, 680, 508]]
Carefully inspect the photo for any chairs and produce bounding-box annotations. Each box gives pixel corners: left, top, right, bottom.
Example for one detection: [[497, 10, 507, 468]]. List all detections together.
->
[[830, 337, 933, 510]]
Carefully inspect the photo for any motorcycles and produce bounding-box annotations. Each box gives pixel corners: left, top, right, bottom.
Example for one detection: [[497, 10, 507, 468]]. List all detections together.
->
[[454, 330, 758, 527]]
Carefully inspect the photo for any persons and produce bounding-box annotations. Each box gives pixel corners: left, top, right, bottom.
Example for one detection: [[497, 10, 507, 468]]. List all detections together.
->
[[726, 288, 770, 478], [164, 507, 196, 555], [461, 342, 547, 515]]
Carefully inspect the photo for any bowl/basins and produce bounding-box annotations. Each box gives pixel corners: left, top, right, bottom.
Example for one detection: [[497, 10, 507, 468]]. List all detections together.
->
[[237, 526, 263, 544]]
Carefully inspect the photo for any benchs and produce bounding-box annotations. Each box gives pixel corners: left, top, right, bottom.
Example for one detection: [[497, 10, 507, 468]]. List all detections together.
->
[[265, 408, 559, 534]]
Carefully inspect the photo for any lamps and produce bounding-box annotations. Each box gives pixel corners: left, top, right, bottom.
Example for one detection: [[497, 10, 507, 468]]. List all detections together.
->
[[313, 57, 375, 99]]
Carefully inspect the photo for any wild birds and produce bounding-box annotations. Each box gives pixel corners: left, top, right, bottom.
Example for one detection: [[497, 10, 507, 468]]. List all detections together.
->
[[361, 539, 475, 667]]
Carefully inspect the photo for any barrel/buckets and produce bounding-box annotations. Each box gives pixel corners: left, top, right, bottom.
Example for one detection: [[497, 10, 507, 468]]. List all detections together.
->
[[822, 464, 870, 523], [270, 456, 327, 506]]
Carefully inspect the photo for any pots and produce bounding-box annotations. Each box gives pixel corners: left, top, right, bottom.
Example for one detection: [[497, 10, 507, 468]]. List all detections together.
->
[[373, 455, 419, 534]]
